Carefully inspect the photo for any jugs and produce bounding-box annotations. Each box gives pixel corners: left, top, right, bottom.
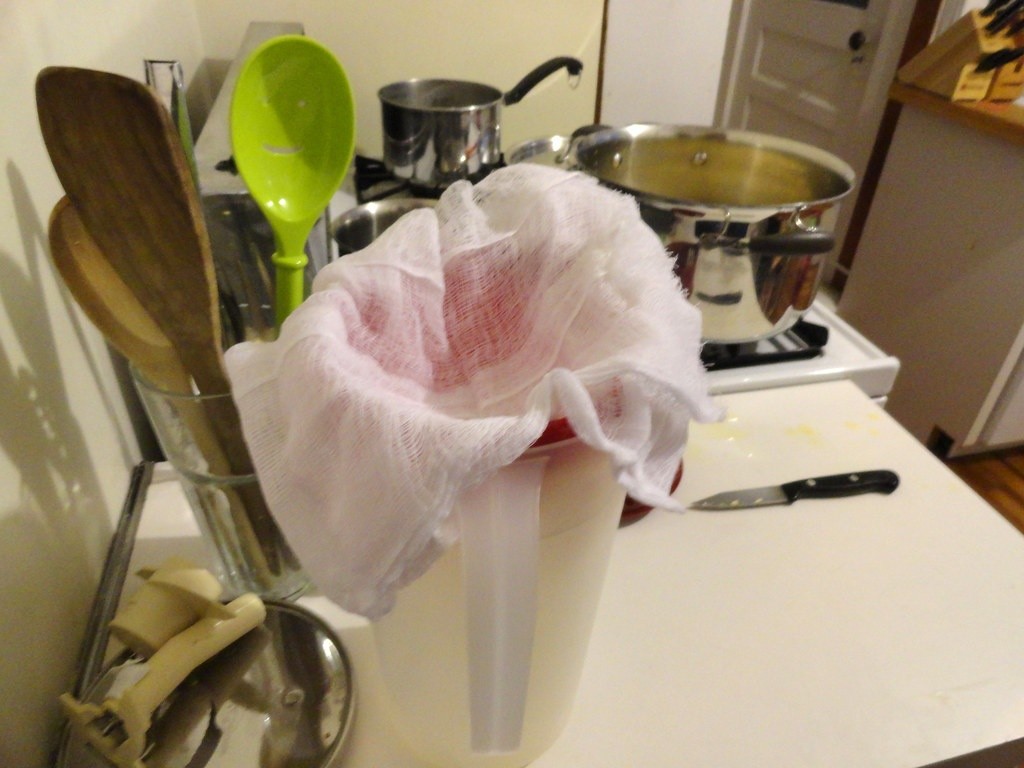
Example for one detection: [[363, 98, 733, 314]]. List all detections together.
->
[[372, 420, 627, 768]]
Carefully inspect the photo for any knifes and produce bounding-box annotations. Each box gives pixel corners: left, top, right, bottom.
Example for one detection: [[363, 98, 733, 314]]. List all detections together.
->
[[690, 470, 899, 510]]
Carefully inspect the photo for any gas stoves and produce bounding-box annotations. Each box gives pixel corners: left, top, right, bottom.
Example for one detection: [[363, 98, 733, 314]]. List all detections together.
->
[[326, 154, 903, 397]]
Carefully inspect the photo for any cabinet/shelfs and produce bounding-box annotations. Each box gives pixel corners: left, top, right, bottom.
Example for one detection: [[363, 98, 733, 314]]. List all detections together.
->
[[835, 82, 1024, 469]]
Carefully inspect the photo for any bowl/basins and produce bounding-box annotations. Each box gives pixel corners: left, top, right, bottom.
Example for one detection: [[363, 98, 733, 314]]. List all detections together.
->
[[329, 198, 439, 257]]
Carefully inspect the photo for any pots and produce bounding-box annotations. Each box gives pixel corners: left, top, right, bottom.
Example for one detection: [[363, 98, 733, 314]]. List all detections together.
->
[[377, 55, 584, 192], [563, 120, 859, 345]]
